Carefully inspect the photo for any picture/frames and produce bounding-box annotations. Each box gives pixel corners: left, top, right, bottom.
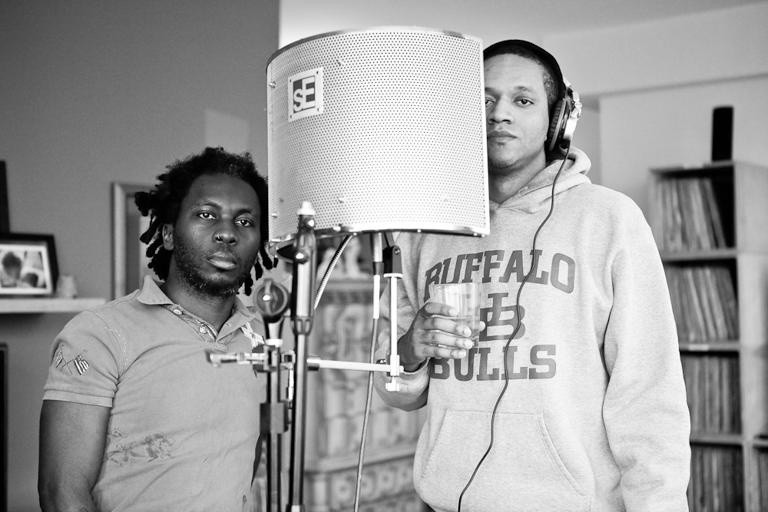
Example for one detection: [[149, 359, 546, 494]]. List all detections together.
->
[[109, 180, 159, 306], [0, 230, 61, 298]]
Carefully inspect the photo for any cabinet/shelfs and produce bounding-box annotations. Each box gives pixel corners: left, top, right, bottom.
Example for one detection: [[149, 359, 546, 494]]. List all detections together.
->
[[637, 160, 768, 512], [298, 233, 438, 512]]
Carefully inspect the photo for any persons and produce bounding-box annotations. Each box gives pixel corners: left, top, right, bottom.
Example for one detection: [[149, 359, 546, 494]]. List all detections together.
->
[[17, 273, 38, 287], [372, 38, 694, 512], [36, 145, 268, 512], [0, 251, 23, 287]]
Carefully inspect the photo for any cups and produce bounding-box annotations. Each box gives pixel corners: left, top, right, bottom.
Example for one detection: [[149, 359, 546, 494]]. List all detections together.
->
[[429, 282, 481, 347]]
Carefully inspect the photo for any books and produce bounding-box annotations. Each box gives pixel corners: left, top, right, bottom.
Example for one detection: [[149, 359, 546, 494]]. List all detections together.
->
[[648, 176, 743, 512], [758, 453, 768, 512]]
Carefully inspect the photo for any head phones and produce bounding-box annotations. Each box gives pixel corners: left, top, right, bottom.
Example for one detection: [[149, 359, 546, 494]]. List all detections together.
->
[[483, 39, 581, 153]]
